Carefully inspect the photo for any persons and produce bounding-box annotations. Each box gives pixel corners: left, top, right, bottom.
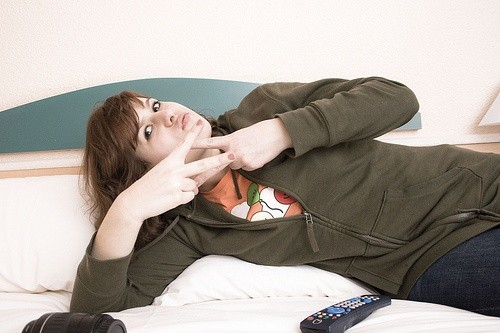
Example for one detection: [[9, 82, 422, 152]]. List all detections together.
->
[[70, 76, 500, 319]]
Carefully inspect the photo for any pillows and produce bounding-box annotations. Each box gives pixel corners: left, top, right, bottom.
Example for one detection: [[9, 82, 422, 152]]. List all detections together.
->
[[149, 254, 372, 307], [0, 175, 101, 295]]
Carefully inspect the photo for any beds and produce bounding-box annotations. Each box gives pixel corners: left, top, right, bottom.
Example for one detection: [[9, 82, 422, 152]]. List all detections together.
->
[[0, 149, 500, 333]]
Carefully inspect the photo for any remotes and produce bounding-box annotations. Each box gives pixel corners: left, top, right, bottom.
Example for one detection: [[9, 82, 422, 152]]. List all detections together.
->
[[300, 295, 391, 333]]
